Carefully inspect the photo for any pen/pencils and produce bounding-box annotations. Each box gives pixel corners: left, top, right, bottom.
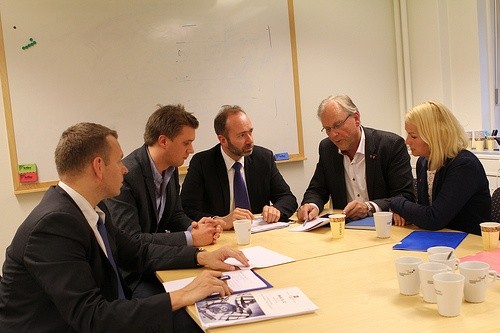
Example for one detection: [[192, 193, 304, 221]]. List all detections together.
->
[[303, 219, 307, 227], [217, 275, 230, 280], [255, 216, 264, 219]]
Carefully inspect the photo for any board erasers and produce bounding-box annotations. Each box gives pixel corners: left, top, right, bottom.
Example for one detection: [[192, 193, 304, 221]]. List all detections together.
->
[[274, 152, 289, 161]]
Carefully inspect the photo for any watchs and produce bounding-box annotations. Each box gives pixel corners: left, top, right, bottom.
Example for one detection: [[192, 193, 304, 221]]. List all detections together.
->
[[365, 202, 375, 216], [195, 247, 208, 268]]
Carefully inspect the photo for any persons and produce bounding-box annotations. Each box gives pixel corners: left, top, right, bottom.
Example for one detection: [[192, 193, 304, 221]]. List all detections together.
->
[[0, 122, 234, 333], [389, 100, 492, 236], [180, 105, 298, 231], [297, 94, 416, 222], [101, 105, 227, 299]]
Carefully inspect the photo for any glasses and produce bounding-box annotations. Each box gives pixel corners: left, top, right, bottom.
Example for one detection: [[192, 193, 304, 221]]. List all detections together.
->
[[320, 114, 352, 135]]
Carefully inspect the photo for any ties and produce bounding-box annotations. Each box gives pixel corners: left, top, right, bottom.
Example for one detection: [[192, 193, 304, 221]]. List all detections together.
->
[[97, 214, 125, 300], [232, 162, 250, 209]]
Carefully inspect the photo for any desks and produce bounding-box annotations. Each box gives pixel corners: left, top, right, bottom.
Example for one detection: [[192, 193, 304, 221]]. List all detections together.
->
[[155, 213, 500, 333]]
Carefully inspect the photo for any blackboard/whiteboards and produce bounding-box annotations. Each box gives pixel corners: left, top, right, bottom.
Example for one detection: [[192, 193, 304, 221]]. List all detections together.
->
[[0, 0, 308, 195]]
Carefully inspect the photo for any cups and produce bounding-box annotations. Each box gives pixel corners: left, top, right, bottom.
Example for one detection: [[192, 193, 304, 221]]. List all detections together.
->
[[429, 253, 461, 272], [431, 272, 465, 316], [459, 261, 497, 303], [373, 211, 393, 238], [466, 137, 473, 150], [233, 219, 251, 244], [395, 256, 422, 297], [486, 136, 496, 151], [475, 137, 485, 150], [479, 222, 500, 250], [420, 262, 452, 303], [427, 246, 455, 254], [329, 213, 347, 239]]
